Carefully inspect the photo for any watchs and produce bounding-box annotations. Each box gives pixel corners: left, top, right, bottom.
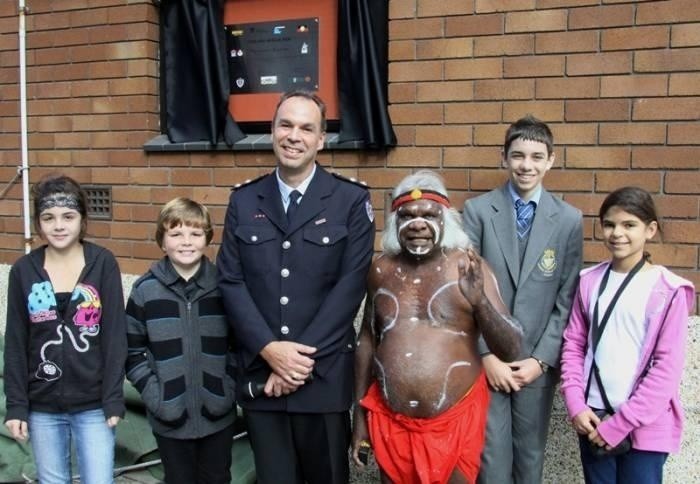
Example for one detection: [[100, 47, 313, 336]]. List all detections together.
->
[[533, 356, 549, 374]]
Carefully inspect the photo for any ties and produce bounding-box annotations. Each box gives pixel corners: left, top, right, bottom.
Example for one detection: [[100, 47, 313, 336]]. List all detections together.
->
[[517, 201, 534, 264], [287, 190, 302, 226]]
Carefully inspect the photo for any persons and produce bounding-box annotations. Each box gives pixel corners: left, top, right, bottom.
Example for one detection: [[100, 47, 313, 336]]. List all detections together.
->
[[218, 90, 375, 483], [3, 172, 129, 484], [457, 114, 583, 484], [348, 171, 523, 484], [120, 197, 241, 484], [566, 186, 696, 484]]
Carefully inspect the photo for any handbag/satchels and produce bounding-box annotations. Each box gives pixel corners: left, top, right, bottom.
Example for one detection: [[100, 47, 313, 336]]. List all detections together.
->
[[585, 409, 631, 456]]
